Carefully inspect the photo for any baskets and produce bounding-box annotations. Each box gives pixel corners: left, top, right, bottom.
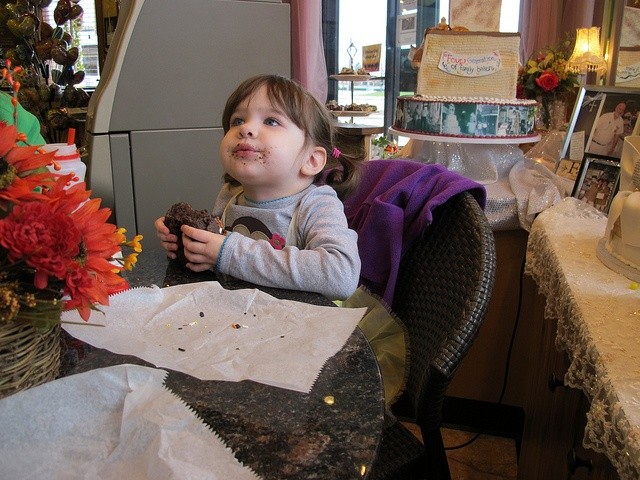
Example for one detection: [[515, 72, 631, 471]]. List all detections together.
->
[[0, 300, 62, 399]]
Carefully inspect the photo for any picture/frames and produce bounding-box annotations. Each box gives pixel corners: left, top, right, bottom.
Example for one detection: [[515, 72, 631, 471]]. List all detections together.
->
[[574, 157, 621, 217], [583, 92, 640, 160]]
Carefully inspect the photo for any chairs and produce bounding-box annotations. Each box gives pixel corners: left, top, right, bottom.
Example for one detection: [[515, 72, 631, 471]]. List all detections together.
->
[[323, 158, 497, 476]]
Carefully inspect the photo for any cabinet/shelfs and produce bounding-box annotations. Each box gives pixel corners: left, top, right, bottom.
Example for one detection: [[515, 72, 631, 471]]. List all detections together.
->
[[519, 208, 640, 479], [429, 223, 542, 449]]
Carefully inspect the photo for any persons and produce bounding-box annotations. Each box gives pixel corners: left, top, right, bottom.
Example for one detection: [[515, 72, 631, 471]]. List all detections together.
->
[[579, 174, 617, 210], [623, 111, 631, 133], [420, 105, 428, 131], [461, 110, 484, 133], [414, 104, 421, 130], [507, 106, 524, 134], [589, 102, 626, 156], [154, 73, 367, 308]]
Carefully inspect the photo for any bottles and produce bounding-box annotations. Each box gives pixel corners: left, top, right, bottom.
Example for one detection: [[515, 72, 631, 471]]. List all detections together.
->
[[41, 142, 87, 206]]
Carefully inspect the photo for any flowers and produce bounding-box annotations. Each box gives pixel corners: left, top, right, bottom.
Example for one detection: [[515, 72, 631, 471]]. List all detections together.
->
[[518, 34, 576, 139], [0, 63, 143, 323]]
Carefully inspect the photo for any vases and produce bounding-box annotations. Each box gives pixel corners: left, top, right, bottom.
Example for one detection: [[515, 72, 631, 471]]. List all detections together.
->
[[2, 283, 67, 402]]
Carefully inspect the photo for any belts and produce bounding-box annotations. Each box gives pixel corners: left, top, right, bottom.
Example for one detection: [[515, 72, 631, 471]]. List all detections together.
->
[[592, 138, 606, 146]]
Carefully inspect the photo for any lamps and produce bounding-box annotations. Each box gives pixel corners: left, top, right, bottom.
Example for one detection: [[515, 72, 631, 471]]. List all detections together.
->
[[565, 29, 610, 114]]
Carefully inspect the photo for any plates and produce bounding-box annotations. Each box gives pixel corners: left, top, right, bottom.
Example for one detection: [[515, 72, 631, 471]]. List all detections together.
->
[[329, 74, 370, 81], [331, 110, 370, 116]]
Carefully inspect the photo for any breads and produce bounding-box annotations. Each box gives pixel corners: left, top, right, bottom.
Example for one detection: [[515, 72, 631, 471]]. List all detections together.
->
[[165, 202, 223, 270]]
